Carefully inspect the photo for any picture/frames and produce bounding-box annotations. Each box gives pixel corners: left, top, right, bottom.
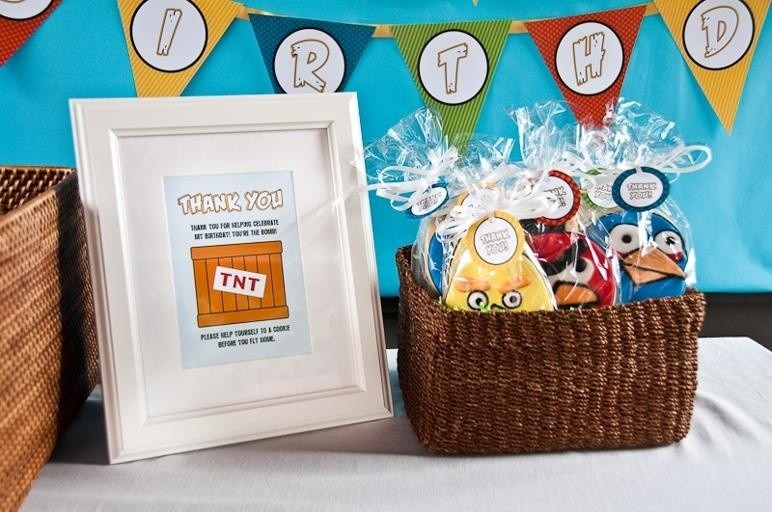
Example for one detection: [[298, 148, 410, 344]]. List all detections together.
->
[[69, 92, 394, 466]]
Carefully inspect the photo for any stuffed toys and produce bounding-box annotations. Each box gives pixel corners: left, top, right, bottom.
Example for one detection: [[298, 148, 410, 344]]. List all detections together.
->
[[423, 166, 690, 312]]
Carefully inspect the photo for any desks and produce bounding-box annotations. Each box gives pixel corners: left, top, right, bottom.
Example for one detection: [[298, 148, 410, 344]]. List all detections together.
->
[[18, 337, 772, 512]]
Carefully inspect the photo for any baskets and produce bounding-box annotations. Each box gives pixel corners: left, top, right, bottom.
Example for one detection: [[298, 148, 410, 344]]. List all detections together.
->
[[0, 165, 101, 512], [395, 244, 707, 456]]
